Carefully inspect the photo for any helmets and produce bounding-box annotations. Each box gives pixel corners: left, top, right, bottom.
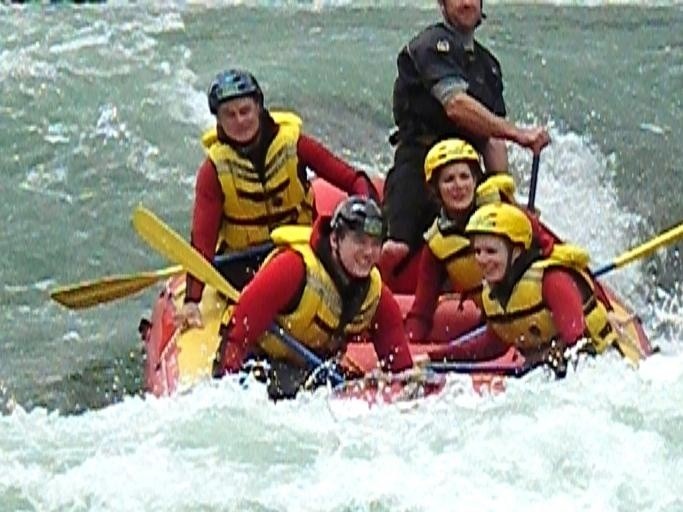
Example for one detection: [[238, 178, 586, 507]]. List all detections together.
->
[[464, 201, 533, 250], [208, 67, 258, 114], [423, 138, 479, 184], [330, 193, 388, 237]]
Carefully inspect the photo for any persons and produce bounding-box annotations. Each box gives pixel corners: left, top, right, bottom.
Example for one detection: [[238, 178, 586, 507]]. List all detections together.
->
[[180, 69, 382, 329], [404, 139, 558, 343], [379, 0, 551, 283], [409, 201, 622, 358], [212, 196, 412, 397]]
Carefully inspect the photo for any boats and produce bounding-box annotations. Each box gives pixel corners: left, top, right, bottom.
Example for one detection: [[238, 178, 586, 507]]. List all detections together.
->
[[140, 173, 658, 409]]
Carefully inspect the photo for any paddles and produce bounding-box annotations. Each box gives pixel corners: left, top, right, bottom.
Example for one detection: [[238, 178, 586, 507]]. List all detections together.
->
[[51, 242, 276, 310], [451, 224, 682, 344], [131, 206, 345, 384]]
